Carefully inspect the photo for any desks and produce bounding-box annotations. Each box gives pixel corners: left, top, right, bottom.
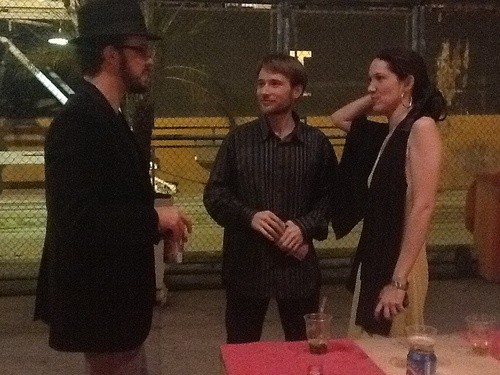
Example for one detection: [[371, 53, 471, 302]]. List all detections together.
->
[[219, 328, 500, 375]]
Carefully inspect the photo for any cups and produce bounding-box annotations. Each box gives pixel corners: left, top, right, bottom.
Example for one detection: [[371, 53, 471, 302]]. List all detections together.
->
[[464, 313, 495, 356], [405, 324, 438, 352], [304, 313, 332, 355]]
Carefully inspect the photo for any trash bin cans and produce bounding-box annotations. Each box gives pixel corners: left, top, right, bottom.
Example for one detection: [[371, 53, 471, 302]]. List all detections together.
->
[[464, 172, 500, 281]]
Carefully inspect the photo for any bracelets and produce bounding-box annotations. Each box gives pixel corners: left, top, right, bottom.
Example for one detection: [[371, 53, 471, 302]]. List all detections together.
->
[[393, 280, 408, 290]]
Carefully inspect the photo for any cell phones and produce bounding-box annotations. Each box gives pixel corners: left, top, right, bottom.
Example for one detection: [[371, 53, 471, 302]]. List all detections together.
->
[[374, 291, 409, 321]]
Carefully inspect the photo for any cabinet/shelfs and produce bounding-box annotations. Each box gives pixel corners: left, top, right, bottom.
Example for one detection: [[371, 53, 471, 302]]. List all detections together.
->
[[154, 182, 179, 301], [473, 174, 500, 283]]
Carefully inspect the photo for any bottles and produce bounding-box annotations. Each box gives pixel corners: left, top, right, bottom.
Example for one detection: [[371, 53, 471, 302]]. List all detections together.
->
[[308, 364, 323, 375]]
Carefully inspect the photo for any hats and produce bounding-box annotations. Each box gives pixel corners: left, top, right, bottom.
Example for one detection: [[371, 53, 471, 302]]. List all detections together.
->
[[68, 0, 163, 42]]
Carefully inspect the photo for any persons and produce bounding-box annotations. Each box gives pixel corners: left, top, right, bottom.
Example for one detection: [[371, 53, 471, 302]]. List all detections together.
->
[[34, 0, 191, 375], [204, 55, 338, 344], [332, 47, 447, 337]]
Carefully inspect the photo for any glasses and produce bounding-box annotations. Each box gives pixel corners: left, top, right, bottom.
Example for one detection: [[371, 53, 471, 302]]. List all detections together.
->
[[117, 45, 152, 57]]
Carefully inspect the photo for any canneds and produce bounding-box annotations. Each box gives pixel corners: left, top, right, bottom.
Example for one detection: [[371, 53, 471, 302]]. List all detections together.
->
[[163, 232, 184, 264], [405, 349, 437, 375]]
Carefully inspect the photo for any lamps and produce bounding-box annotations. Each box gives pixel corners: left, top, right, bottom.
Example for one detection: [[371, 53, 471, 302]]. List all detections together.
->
[[48, 22, 71, 45]]
